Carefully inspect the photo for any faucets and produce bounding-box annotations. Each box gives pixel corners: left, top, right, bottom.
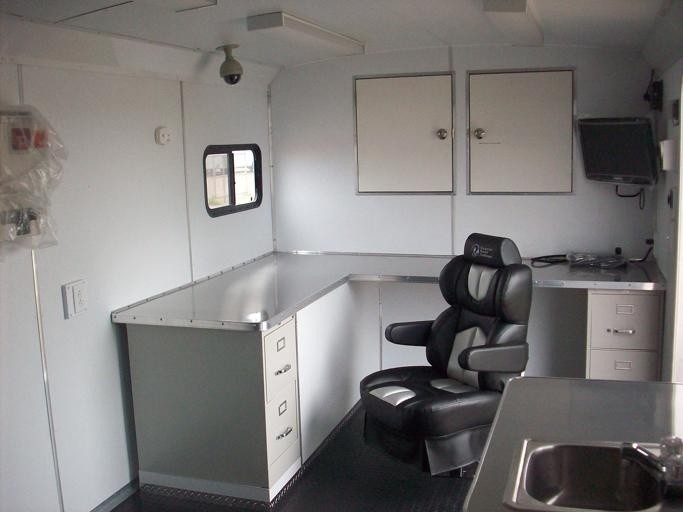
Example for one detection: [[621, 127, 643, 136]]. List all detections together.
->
[[621, 433, 683, 489]]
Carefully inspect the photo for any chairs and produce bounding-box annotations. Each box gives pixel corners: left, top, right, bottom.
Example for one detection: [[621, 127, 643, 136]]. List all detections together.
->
[[360, 232, 533, 476]]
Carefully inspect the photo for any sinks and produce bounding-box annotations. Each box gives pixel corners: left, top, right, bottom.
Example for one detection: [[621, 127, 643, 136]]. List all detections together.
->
[[504, 436, 682, 511]]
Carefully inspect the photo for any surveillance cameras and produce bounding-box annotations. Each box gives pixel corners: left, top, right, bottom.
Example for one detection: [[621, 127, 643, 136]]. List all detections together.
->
[[220, 59, 243, 84]]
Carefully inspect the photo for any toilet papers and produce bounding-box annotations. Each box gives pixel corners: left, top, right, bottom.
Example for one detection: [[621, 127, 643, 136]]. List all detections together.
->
[[658, 138, 676, 171]]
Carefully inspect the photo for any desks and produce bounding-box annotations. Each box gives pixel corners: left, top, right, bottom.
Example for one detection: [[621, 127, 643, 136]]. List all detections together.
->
[[110, 251, 666, 508]]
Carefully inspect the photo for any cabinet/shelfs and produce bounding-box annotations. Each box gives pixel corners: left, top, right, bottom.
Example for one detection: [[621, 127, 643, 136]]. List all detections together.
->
[[465, 67, 577, 196], [352, 70, 456, 196]]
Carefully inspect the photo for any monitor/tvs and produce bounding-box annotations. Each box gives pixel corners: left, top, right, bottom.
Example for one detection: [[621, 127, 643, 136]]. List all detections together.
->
[[579, 117, 661, 186]]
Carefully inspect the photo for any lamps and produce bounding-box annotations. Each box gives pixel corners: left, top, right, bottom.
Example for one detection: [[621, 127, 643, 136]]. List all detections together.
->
[[247, 12, 365, 57], [483, 0, 545, 47]]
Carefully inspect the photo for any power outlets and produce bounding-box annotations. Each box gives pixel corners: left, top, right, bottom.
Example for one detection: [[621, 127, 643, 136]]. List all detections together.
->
[[653, 232, 658, 258]]
